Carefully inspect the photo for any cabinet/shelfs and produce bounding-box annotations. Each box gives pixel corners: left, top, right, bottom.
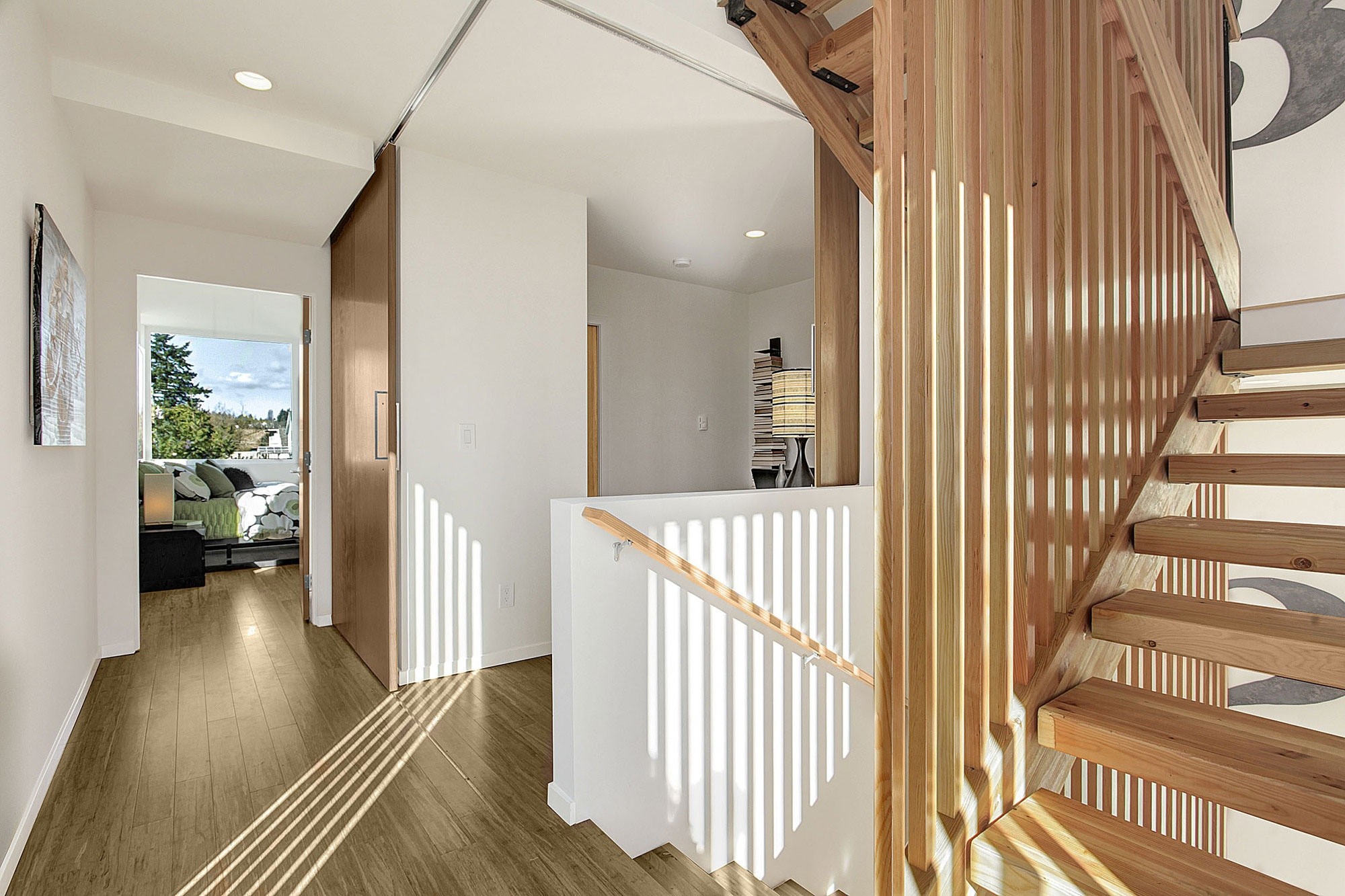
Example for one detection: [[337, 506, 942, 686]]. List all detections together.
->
[[751, 337, 781, 489]]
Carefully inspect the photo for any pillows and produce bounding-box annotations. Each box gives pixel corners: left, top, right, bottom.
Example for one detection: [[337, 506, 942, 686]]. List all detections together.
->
[[165, 465, 211, 502], [165, 461, 196, 474], [138, 460, 165, 474], [206, 458, 223, 471], [224, 468, 255, 490], [196, 462, 235, 498], [139, 463, 176, 503]]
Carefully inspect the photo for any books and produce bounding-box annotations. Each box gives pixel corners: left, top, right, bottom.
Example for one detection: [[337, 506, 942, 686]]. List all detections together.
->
[[173, 519, 203, 526], [752, 357, 787, 469]]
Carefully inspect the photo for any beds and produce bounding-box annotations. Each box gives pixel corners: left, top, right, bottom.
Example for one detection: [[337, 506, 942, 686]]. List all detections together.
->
[[139, 481, 299, 573]]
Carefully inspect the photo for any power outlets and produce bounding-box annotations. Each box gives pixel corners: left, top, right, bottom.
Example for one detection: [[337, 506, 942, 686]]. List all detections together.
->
[[499, 583, 514, 610]]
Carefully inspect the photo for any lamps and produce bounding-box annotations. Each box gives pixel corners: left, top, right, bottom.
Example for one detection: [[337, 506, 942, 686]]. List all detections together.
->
[[143, 475, 175, 522], [773, 365, 815, 487]]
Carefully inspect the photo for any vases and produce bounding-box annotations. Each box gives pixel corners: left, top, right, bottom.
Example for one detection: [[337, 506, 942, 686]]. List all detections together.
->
[[775, 463, 788, 488]]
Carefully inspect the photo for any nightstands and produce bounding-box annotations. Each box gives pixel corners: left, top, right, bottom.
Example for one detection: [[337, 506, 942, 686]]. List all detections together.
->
[[139, 523, 206, 593]]
